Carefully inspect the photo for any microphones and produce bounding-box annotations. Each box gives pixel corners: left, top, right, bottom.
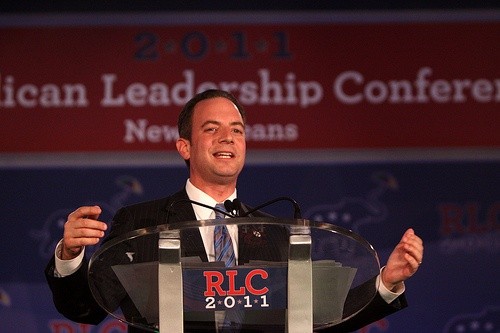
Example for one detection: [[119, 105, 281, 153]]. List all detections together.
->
[[163, 200, 235, 223], [233, 197, 303, 219]]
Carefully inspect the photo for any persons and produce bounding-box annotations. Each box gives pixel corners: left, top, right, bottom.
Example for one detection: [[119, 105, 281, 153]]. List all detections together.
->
[[44, 89, 424, 332]]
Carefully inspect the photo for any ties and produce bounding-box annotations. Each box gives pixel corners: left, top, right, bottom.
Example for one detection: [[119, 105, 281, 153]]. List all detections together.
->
[[213, 202, 244, 332]]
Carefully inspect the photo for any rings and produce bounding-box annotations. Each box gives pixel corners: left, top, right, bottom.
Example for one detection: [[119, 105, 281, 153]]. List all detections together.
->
[[417, 260, 423, 266]]
